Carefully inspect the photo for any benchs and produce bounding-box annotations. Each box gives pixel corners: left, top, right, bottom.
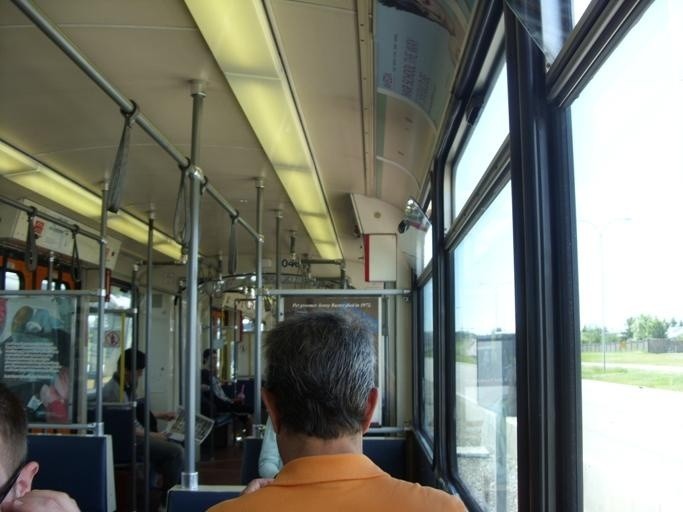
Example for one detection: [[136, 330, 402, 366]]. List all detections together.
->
[[86, 400, 157, 511], [166, 394, 411, 512], [28, 433, 118, 511]]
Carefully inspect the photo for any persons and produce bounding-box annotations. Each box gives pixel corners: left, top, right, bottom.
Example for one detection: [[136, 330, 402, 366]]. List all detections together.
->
[[204, 305, 470, 512], [201, 346, 248, 443], [98, 347, 183, 510], [0, 383, 82, 511]]
